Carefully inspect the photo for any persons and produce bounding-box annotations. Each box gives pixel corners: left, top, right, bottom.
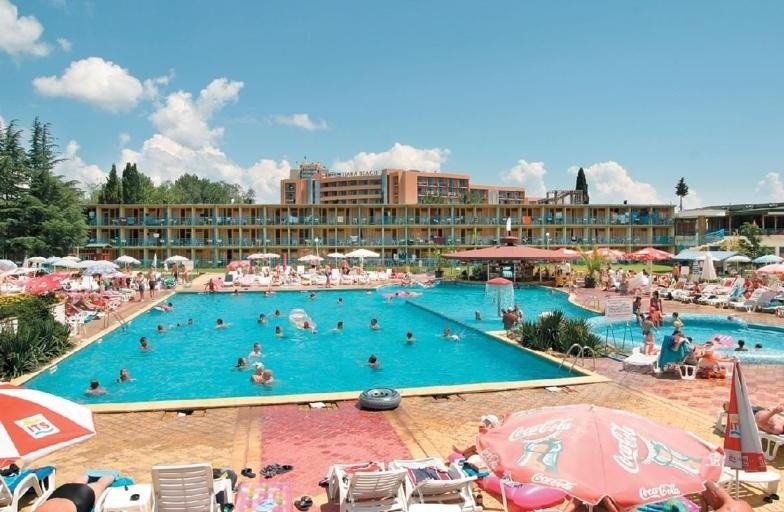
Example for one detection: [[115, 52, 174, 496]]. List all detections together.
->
[[0, 239, 783, 511]]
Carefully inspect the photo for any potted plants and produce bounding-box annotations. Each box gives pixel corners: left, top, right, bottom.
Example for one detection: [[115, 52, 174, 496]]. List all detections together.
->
[[430, 250, 447, 279], [572, 240, 607, 289]]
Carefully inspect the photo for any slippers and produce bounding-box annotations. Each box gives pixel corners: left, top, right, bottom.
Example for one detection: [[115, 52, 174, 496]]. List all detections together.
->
[[295, 501, 308, 511], [763, 497, 772, 503], [301, 496, 312, 506], [770, 494, 779, 500], [241, 468, 255, 478]]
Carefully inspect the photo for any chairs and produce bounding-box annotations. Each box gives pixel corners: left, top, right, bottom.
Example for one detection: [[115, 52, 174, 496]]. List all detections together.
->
[[0, 271, 194, 337], [219, 260, 394, 288], [712, 408, 783, 462], [1, 459, 237, 512], [317, 456, 484, 512], [622, 338, 736, 381], [598, 259, 784, 317]]
